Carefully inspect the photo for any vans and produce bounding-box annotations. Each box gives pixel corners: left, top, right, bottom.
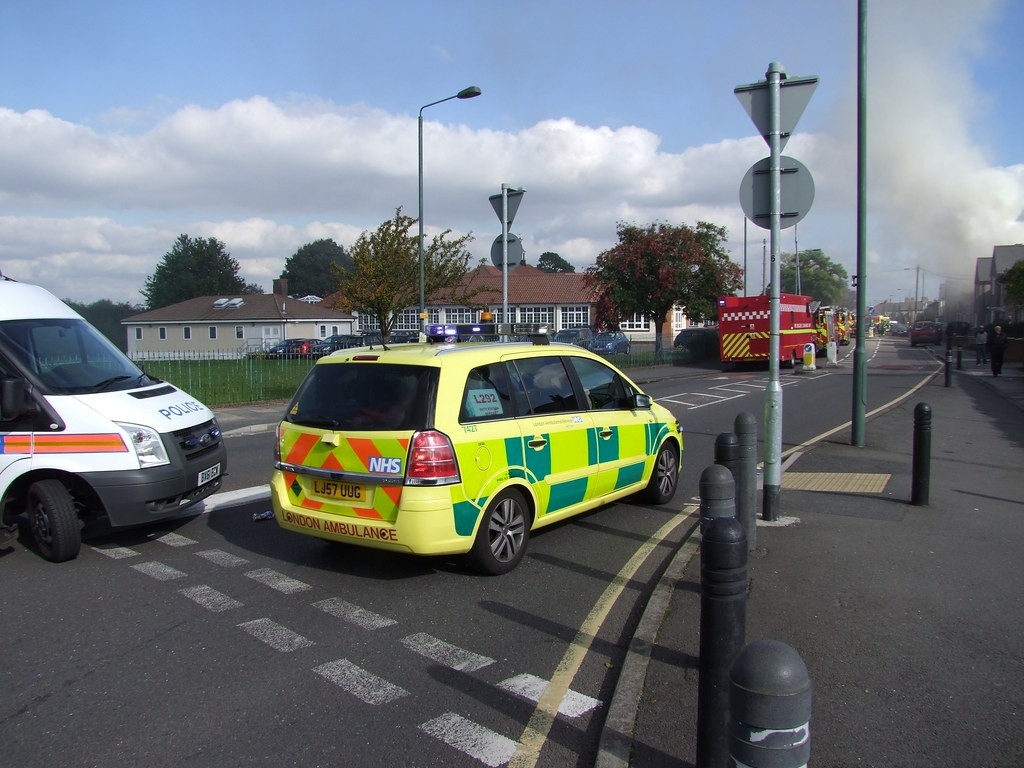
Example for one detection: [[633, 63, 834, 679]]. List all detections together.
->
[[0, 272, 231, 565]]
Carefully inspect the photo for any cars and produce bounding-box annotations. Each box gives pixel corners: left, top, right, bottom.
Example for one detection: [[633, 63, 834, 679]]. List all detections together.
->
[[552, 327, 595, 349], [264, 330, 420, 359], [890, 323, 911, 338], [587, 330, 632, 356], [908, 320, 942, 348]]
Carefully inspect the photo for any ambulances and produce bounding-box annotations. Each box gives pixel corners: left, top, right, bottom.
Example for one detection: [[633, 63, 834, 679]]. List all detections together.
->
[[269, 310, 684, 576]]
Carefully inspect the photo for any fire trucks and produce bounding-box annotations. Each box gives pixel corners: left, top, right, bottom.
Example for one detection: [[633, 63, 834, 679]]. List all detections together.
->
[[674, 292, 892, 369]]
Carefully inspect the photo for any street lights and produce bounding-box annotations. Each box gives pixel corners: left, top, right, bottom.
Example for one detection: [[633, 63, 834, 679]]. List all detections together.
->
[[417, 84, 483, 342], [794, 248, 823, 295], [903, 266, 926, 311]]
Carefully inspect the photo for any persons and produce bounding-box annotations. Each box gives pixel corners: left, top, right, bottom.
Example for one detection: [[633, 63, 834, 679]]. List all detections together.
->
[[1005, 314, 1014, 336], [988, 326, 1007, 378], [869, 318, 888, 337], [975, 325, 989, 367]]
